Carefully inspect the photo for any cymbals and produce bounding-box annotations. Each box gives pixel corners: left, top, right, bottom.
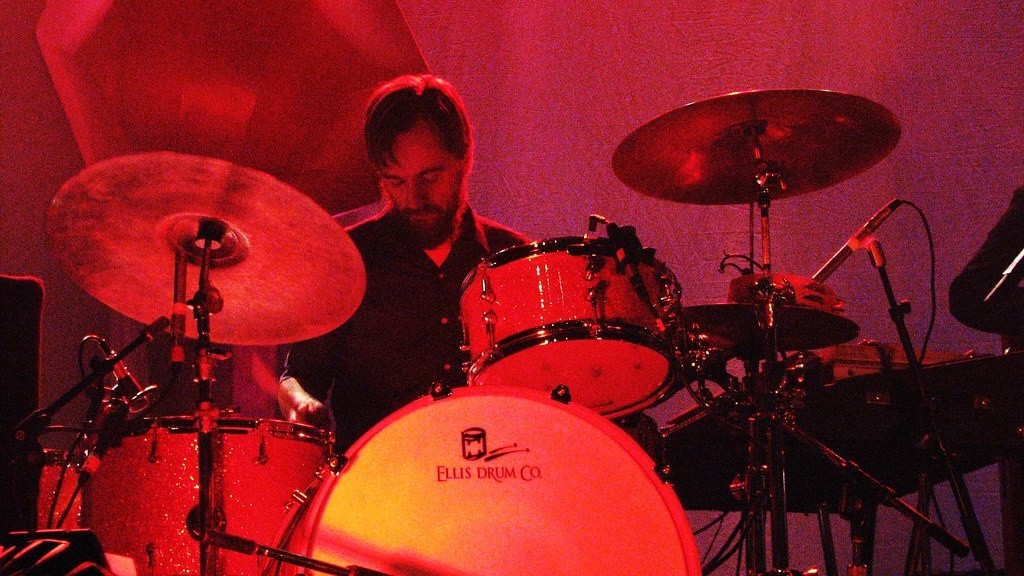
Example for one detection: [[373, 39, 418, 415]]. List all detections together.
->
[[678, 301, 860, 354], [45, 151, 368, 346], [730, 272, 847, 316], [609, 86, 903, 207]]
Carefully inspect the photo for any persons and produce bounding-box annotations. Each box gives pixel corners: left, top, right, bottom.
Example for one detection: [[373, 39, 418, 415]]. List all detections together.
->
[[276, 71, 542, 454], [948, 184, 1024, 341]]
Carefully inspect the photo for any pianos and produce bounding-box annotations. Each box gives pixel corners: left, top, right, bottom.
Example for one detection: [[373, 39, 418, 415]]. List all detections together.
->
[[658, 350, 1024, 513]]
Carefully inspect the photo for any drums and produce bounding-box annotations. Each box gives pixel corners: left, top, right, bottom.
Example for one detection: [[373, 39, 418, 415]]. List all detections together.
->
[[457, 234, 682, 421], [86, 413, 334, 576], [261, 381, 703, 576], [38, 448, 83, 531]]
